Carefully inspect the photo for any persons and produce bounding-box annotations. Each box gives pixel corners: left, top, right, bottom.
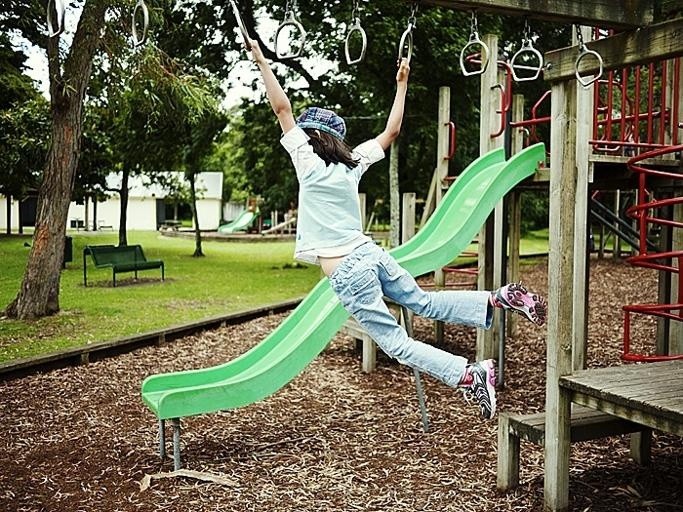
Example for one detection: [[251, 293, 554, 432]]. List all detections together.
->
[[243, 36, 548, 421]]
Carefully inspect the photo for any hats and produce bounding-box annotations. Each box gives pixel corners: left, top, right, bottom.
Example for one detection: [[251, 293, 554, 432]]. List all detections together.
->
[[282, 107, 346, 142]]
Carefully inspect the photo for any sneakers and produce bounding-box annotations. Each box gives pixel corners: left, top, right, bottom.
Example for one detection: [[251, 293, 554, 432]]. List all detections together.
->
[[494, 283, 548, 326], [455, 359, 497, 420]]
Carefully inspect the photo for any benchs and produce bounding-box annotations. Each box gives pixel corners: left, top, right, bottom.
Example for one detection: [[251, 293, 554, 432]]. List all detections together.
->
[[83, 245, 165, 288]]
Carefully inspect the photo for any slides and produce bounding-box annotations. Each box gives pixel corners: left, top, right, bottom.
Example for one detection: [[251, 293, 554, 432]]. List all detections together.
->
[[142, 142, 545, 421]]
